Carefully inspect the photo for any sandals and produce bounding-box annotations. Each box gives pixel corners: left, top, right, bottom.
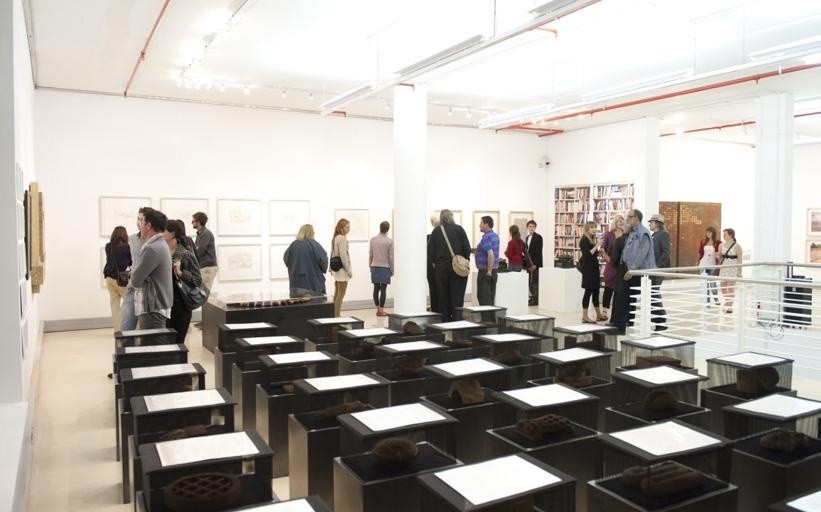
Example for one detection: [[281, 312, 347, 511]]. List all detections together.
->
[[583, 312, 608, 324]]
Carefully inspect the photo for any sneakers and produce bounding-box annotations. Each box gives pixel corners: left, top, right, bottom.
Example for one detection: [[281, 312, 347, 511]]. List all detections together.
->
[[376, 311, 389, 316], [529, 299, 537, 305]]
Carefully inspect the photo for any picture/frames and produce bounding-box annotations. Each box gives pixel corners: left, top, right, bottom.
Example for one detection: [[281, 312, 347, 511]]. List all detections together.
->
[[472, 211, 499, 249], [269, 243, 290, 280], [433, 210, 461, 227], [508, 211, 534, 244], [806, 208, 821, 238], [216, 244, 263, 282], [217, 199, 261, 237], [100, 247, 107, 289], [268, 199, 311, 236], [160, 198, 209, 237], [805, 239, 821, 264], [99, 197, 152, 237], [334, 209, 369, 242]]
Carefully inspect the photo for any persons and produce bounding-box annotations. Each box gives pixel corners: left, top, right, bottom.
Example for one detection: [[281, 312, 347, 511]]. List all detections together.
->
[[330, 218, 352, 317], [524, 220, 543, 305], [699, 227, 722, 308], [368, 221, 394, 315], [601, 209, 670, 335], [716, 228, 742, 313], [103, 207, 218, 344], [476, 216, 499, 305], [283, 224, 328, 297], [505, 225, 525, 272], [427, 209, 471, 322], [580, 221, 608, 324]]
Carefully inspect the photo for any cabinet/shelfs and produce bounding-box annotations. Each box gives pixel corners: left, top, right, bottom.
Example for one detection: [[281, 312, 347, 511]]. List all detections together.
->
[[554, 183, 634, 278]]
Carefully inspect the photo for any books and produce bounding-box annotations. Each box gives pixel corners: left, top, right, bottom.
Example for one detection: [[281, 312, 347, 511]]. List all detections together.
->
[[554, 187, 590, 268], [594, 184, 634, 276]]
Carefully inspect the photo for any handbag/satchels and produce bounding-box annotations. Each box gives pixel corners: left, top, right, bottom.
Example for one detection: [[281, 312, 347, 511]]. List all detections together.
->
[[176, 277, 210, 309], [452, 256, 470, 277], [715, 268, 720, 276], [522, 253, 536, 273], [329, 256, 344, 271], [117, 271, 131, 287]]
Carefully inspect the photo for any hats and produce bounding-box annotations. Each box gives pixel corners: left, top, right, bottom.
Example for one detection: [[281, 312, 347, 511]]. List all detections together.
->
[[648, 214, 665, 224]]
